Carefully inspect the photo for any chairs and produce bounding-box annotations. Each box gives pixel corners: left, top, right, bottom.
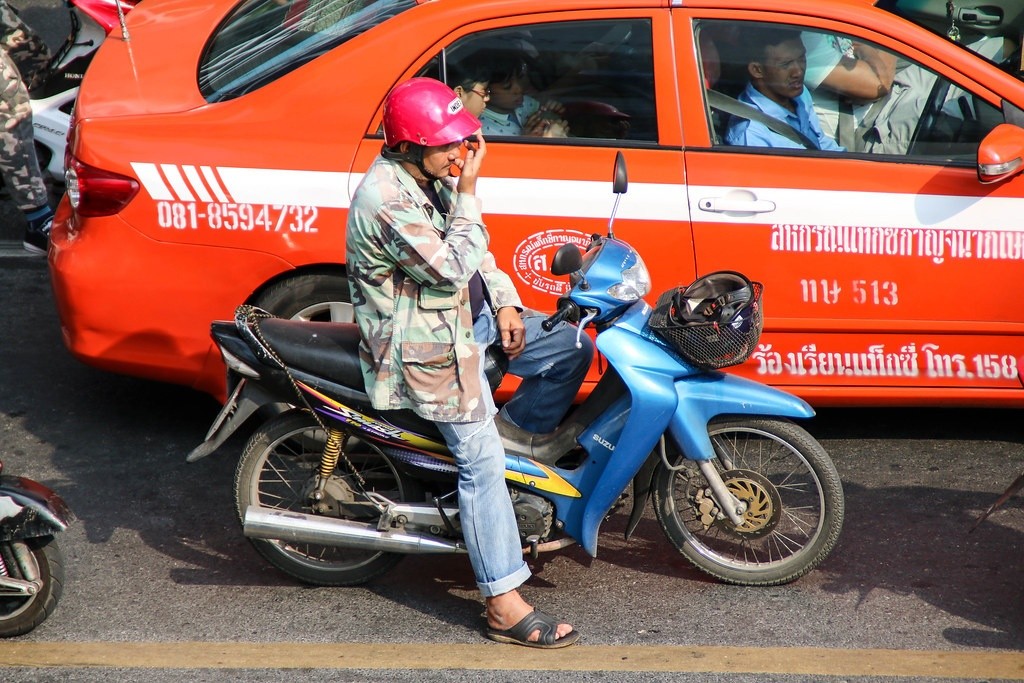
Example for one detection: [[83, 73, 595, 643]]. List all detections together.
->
[[699, 29, 730, 146]]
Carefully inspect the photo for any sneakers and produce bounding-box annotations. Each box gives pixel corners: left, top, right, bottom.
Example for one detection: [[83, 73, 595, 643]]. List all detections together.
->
[[23, 214, 55, 257]]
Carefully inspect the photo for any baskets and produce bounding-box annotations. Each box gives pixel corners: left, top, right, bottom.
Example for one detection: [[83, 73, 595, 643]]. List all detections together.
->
[[649, 281, 764, 369]]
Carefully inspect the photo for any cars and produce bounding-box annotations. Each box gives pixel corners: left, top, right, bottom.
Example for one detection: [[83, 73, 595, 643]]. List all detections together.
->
[[49, 0, 1024, 410]]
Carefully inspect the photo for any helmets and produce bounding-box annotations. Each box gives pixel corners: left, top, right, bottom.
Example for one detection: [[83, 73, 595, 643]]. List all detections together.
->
[[562, 101, 630, 128], [382, 78, 482, 150], [669, 270, 755, 361]]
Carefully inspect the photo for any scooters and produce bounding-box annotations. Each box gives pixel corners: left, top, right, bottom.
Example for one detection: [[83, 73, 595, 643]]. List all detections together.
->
[[0, 0, 143, 202], [0, 455, 74, 640]]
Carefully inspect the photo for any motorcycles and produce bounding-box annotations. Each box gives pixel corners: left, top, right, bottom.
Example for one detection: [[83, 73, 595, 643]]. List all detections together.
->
[[183, 151, 845, 587]]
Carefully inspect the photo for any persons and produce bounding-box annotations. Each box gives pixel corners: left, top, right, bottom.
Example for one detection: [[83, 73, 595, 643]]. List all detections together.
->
[[0, 0, 56, 258], [562, 98, 633, 138], [436, 51, 581, 138], [346, 78, 595, 645], [724, 26, 1019, 156]]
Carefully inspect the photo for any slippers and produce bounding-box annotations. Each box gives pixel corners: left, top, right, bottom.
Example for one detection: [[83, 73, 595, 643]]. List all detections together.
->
[[486, 607, 579, 649]]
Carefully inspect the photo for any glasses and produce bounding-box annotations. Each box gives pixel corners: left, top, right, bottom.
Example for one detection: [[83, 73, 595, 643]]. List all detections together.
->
[[471, 86, 492, 97]]
